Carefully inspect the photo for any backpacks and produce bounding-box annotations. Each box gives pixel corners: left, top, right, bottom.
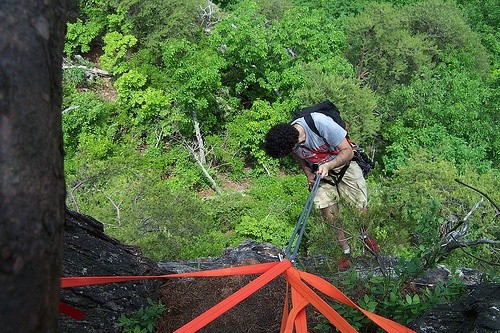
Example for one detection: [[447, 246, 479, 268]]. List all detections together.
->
[[289, 100, 350, 147]]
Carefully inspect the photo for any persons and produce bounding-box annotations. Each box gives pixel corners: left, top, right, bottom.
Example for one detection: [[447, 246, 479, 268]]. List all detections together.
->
[[264, 111, 379, 271]]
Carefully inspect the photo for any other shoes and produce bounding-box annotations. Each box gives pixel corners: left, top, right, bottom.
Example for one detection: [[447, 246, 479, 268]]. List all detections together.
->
[[362, 237, 378, 255], [339, 247, 354, 272]]
